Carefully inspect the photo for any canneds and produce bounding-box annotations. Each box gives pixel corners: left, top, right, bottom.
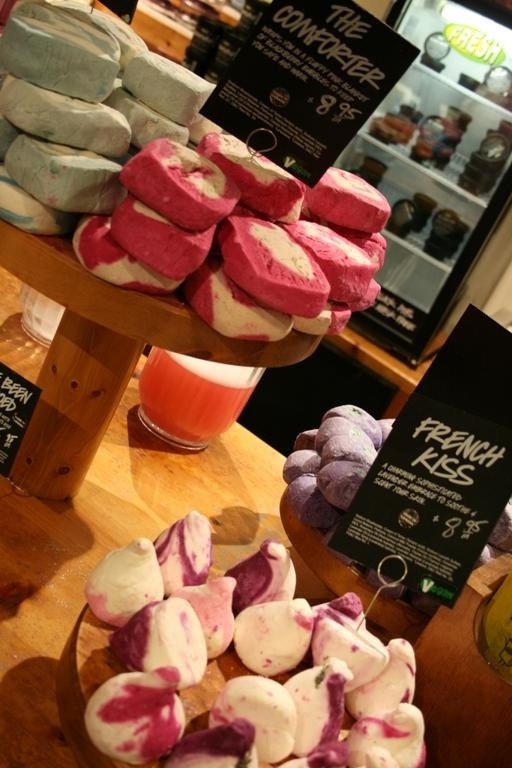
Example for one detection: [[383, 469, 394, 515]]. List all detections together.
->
[[359, 104, 512, 261]]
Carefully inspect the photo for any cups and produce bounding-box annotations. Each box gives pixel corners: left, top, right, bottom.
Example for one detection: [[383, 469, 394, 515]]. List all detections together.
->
[[135, 347, 267, 452]]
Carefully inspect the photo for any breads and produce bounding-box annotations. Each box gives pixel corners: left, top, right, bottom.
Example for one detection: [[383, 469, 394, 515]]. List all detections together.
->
[[1, 0, 392, 345], [80, 402, 512, 767]]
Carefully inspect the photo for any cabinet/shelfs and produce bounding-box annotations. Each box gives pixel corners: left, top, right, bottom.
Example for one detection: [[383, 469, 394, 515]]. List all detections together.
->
[[0, 211, 512, 767]]
